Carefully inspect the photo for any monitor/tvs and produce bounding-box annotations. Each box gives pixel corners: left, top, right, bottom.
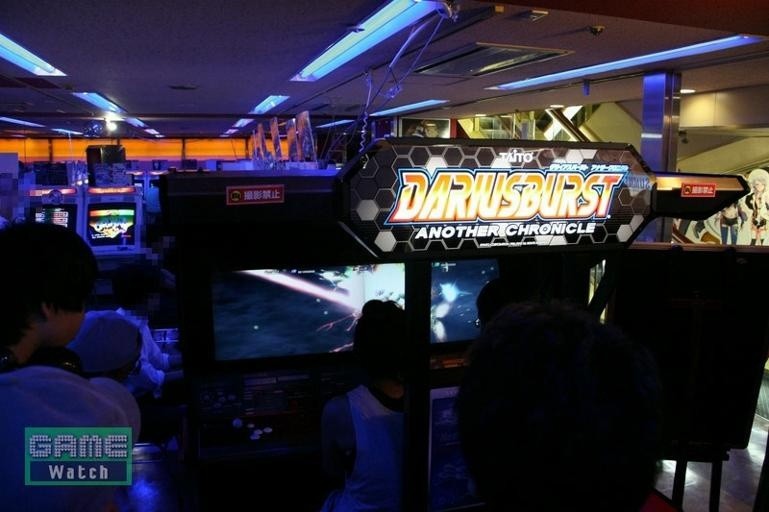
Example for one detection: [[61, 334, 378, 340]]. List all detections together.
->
[[84, 202, 136, 248], [203, 262, 406, 366], [426, 253, 524, 348], [33, 205, 69, 223]]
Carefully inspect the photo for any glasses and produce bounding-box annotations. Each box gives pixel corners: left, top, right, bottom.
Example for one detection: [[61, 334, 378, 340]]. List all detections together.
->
[[84, 286, 99, 307], [128, 359, 142, 377]]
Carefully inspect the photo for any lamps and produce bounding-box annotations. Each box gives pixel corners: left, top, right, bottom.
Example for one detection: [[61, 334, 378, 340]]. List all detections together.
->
[[0, 1, 765, 141]]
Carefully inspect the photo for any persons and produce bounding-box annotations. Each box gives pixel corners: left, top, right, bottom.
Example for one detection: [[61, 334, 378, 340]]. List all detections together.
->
[[719, 200, 747, 244], [743, 169, 769, 245], [68, 313, 144, 384], [113, 254, 186, 443], [309, 300, 414, 511], [455, 272, 662, 511], [1, 363, 142, 512], [0, 222, 87, 379]]
